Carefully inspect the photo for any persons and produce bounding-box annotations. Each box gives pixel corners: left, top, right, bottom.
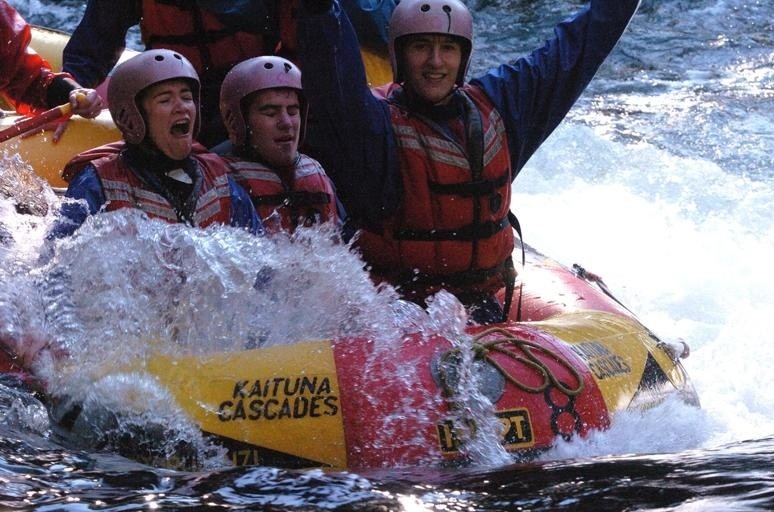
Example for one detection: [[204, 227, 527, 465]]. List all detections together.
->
[[36, 47, 274, 378], [15, 0, 299, 141], [209, 56, 350, 249], [295, 0, 642, 324], [0, 0, 105, 121]]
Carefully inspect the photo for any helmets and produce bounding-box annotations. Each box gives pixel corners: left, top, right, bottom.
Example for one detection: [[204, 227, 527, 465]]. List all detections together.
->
[[219, 56, 311, 146], [108, 49, 202, 143], [388, 0, 474, 86]]
